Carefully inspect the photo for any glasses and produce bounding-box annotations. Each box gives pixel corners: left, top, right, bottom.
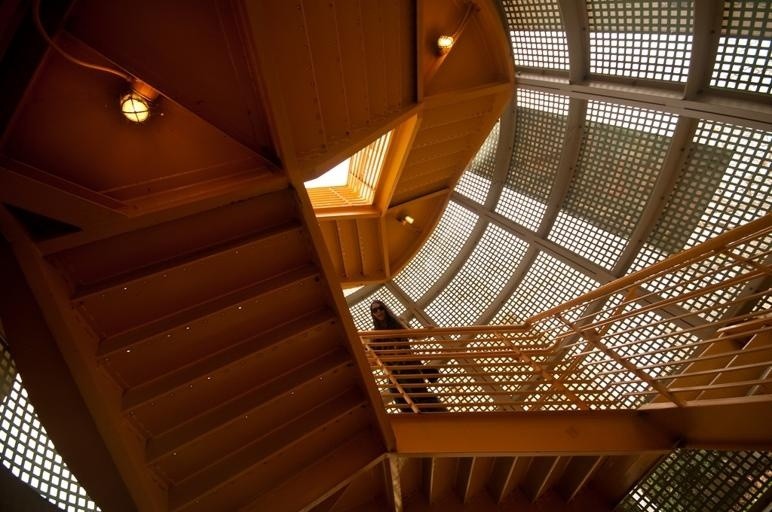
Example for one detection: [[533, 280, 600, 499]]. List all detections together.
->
[[373, 305, 384, 313]]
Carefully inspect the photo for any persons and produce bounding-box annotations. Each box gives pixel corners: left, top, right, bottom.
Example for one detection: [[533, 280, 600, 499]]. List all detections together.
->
[[364, 299, 450, 413]]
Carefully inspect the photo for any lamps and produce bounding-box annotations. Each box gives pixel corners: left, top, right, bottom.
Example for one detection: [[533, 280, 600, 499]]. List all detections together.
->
[[37, 20, 160, 124], [437, 0, 474, 49], [402, 216, 413, 226]]
